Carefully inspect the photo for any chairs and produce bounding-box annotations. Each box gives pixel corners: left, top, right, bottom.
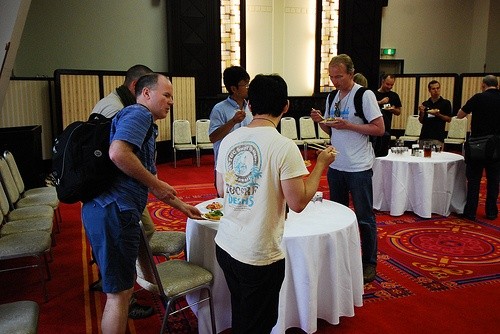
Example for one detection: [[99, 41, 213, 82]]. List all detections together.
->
[[318, 119, 331, 144], [195, 119, 214, 165], [173, 120, 198, 168], [0, 150, 62, 303], [281, 117, 304, 146], [139, 220, 217, 334], [391, 115, 469, 155], [0, 301, 39, 334], [299, 116, 325, 151], [141, 205, 186, 261]]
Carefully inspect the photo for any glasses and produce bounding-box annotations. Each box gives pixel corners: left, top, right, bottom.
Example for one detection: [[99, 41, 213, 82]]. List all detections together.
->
[[238, 82, 250, 88]]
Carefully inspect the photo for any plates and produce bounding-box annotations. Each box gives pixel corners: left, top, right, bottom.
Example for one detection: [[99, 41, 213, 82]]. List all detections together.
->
[[426, 110, 439, 113], [200, 212, 223, 220], [382, 107, 394, 110], [320, 116, 343, 123], [311, 107, 325, 121], [202, 204, 224, 212]]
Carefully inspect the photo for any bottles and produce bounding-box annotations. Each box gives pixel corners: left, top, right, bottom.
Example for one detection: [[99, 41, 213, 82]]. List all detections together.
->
[[415, 149, 422, 157]]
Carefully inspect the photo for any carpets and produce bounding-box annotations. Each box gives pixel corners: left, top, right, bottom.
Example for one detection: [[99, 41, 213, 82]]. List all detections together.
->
[[81, 175, 500, 334]]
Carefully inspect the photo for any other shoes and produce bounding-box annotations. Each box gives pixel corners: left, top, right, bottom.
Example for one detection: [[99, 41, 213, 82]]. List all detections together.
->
[[362, 264, 376, 283], [486, 212, 497, 220], [90, 277, 104, 292], [462, 211, 476, 220], [126, 297, 156, 319]]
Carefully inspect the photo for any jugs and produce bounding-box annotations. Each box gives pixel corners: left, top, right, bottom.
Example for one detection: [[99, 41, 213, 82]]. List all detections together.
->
[[396, 139, 404, 147], [423, 140, 438, 158]]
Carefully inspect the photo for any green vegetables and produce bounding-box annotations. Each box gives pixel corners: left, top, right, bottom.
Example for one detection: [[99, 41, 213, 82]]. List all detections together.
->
[[210, 210, 223, 216]]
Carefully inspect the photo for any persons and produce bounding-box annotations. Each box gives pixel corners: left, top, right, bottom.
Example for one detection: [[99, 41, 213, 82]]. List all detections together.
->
[[458, 75, 500, 219], [209, 66, 253, 197], [418, 81, 452, 151], [81, 73, 204, 334], [91, 63, 155, 318], [370, 75, 402, 157], [213, 74, 337, 334], [353, 73, 367, 87], [311, 54, 385, 281]]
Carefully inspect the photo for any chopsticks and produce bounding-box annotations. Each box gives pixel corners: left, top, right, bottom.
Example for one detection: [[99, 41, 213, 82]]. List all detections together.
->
[[308, 142, 341, 155]]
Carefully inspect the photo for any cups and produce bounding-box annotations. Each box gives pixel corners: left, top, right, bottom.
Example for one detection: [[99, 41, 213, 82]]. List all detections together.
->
[[391, 147, 408, 155], [310, 191, 323, 206], [433, 145, 440, 153]]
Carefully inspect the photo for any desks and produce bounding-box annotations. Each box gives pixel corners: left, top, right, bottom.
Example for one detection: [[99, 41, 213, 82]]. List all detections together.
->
[[186, 197, 364, 334], [372, 152, 467, 219]]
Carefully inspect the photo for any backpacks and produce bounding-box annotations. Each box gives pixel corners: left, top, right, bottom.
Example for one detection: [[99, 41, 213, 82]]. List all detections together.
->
[[328, 86, 389, 157], [52, 103, 159, 204]]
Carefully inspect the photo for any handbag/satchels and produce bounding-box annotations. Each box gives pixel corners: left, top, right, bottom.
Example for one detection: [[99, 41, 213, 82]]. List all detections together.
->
[[465, 134, 496, 161]]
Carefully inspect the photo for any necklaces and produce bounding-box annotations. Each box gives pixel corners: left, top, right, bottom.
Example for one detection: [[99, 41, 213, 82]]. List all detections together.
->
[[252, 117, 275, 127]]
